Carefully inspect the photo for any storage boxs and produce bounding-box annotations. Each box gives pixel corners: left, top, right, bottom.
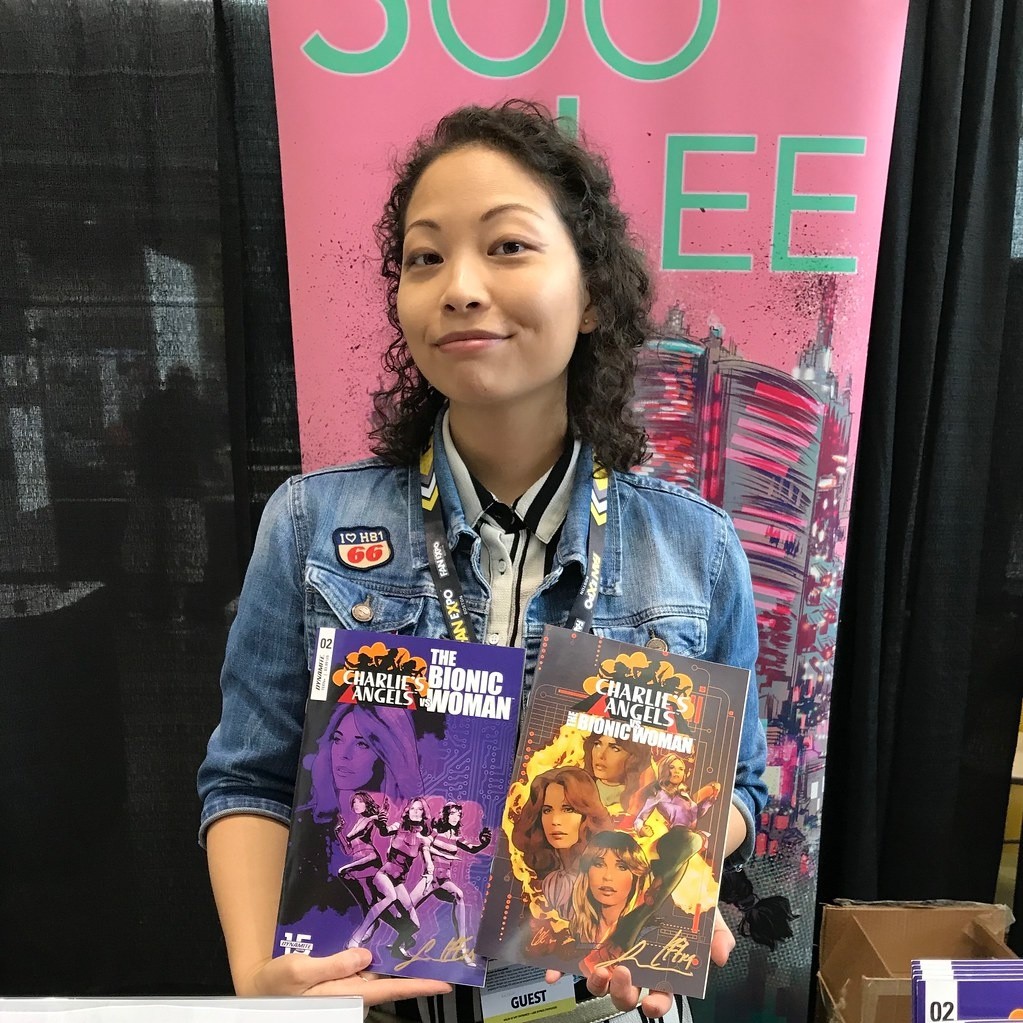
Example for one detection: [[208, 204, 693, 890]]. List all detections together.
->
[[815, 902, 1023, 1023]]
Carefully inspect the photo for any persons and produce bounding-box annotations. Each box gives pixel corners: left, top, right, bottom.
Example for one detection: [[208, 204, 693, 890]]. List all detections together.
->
[[196, 99, 770, 1023]]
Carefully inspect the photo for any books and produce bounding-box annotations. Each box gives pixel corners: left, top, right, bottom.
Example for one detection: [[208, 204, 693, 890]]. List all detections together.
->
[[473, 621, 751, 1000], [271, 628, 528, 990]]
